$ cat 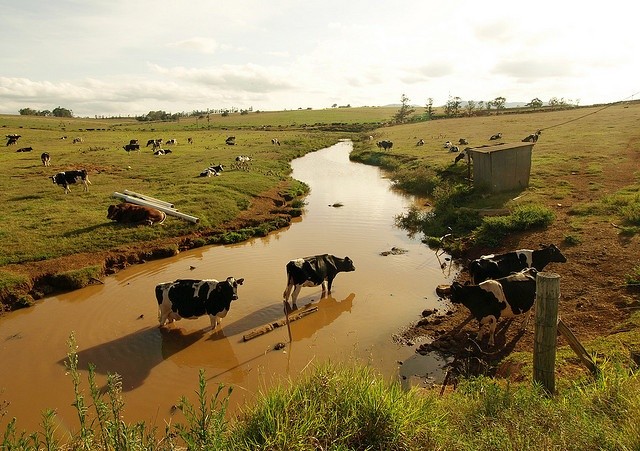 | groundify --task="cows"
[443,140,452,148]
[210,161,227,173]
[122,144,141,156]
[165,139,177,145]
[445,267,539,347]
[49,169,92,194]
[156,139,163,144]
[382,140,394,151]
[236,154,254,165]
[14,135,21,140]
[41,151,51,167]
[200,167,221,178]
[459,138,467,145]
[450,145,459,152]
[469,243,567,286]
[226,136,235,142]
[455,147,473,165]
[271,138,280,147]
[7,139,16,146]
[155,276,244,330]
[153,149,173,156]
[522,134,539,143]
[376,141,382,151]
[130,139,139,145]
[282,254,356,315]
[188,137,193,144]
[17,146,34,152]
[107,202,167,227]
[6,134,15,140]
[146,140,156,147]
[489,133,502,139]
[151,142,160,151]
[417,138,424,146]
[226,141,237,146]
